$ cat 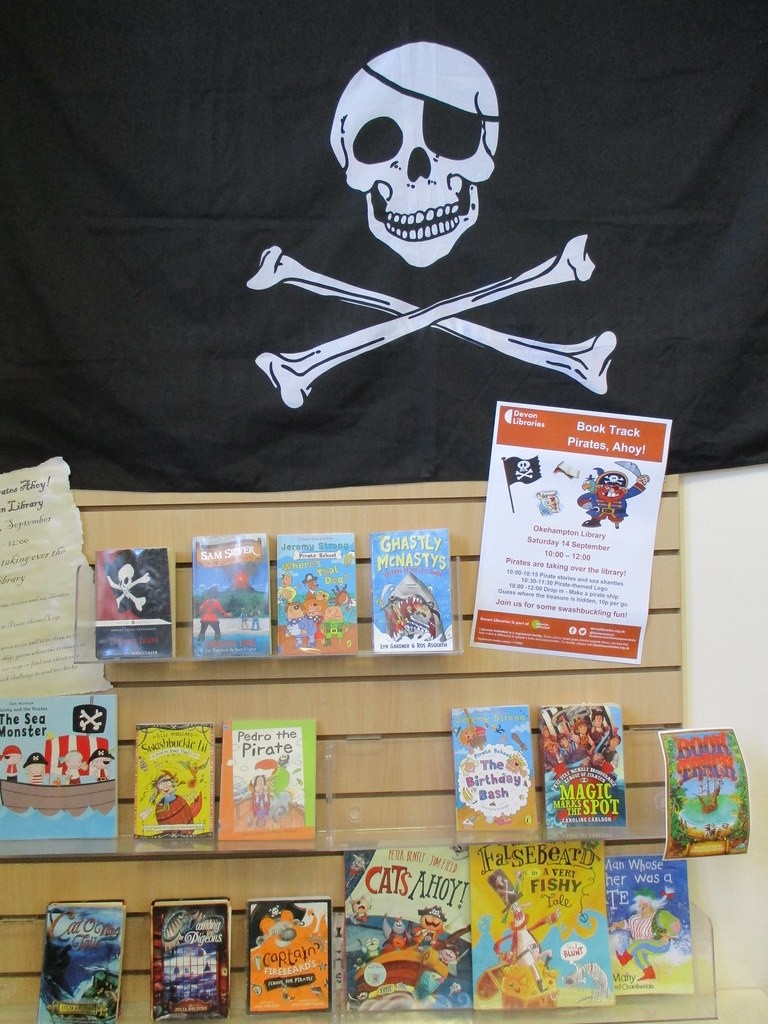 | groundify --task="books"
[133,721,214,840]
[94,547,177,662]
[0,693,119,840]
[658,726,751,862]
[343,846,472,1014]
[191,532,273,658]
[369,528,454,653]
[149,895,232,1021]
[277,533,359,655]
[218,718,315,840]
[469,841,616,1011]
[451,703,539,832]
[35,897,127,1024]
[603,852,694,996]
[245,895,335,1015]
[540,701,628,829]
